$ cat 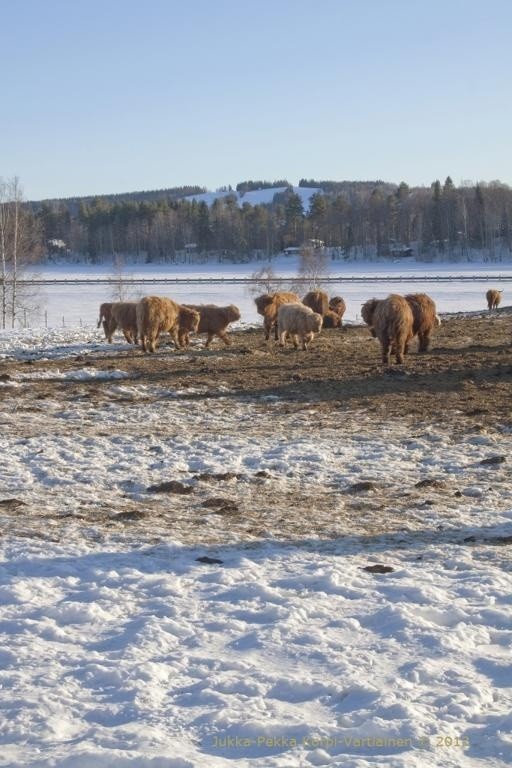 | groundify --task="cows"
[97,302,133,339]
[276,302,323,351]
[373,294,414,365]
[254,291,300,341]
[108,302,138,344]
[302,289,346,328]
[181,303,241,348]
[135,295,200,353]
[486,288,504,311]
[361,293,436,353]
